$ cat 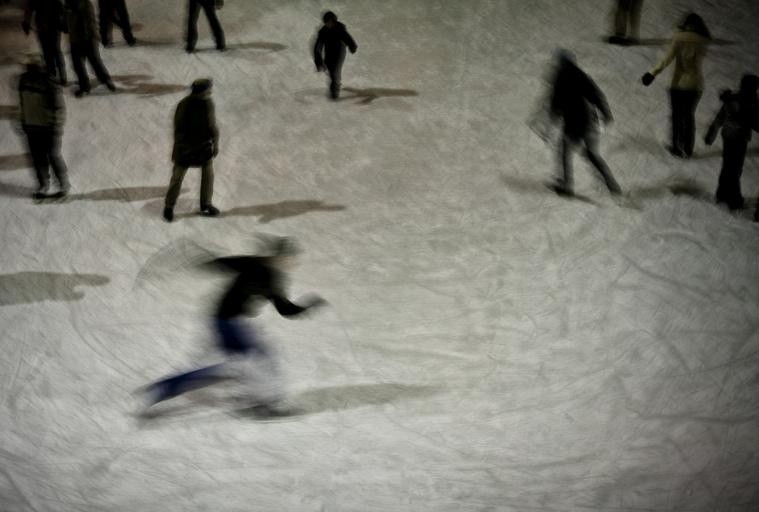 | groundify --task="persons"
[163,79,221,223]
[17,0,138,204]
[539,47,623,201]
[311,11,358,99]
[704,74,758,211]
[184,0,227,52]
[641,14,732,158]
[144,235,331,419]
[605,1,643,47]
